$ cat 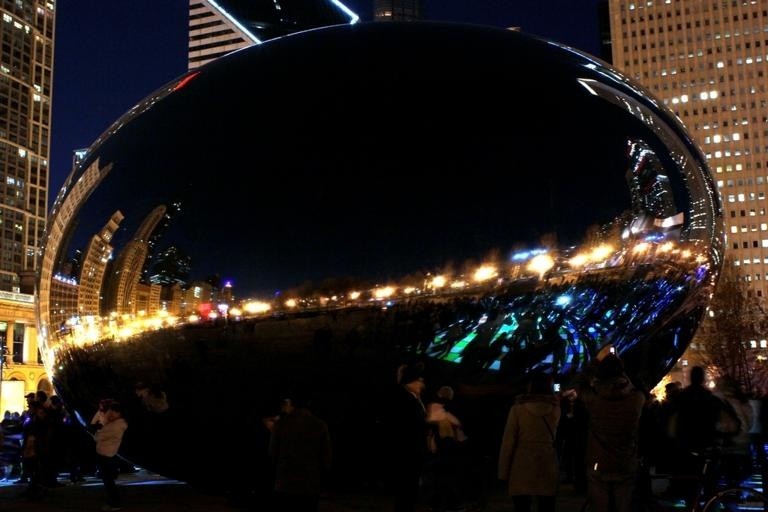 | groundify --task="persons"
[133,380,167,467]
[262,395,326,512]
[382,371,476,512]
[0,390,65,484]
[87,397,128,511]
